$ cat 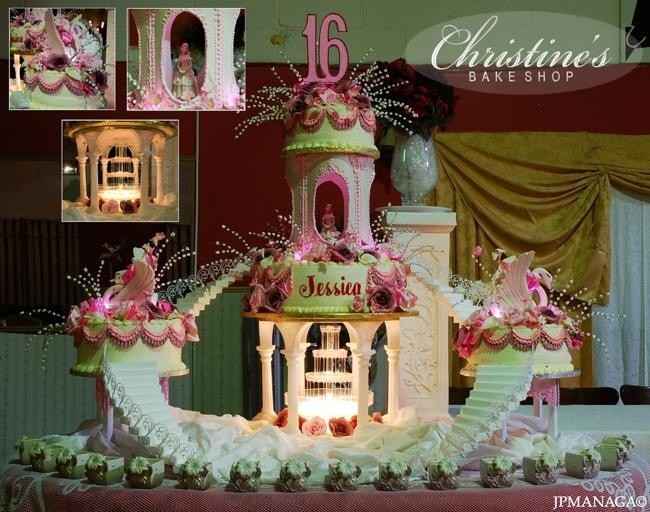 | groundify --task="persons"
[172,42,200,102]
[321,203,342,245]
